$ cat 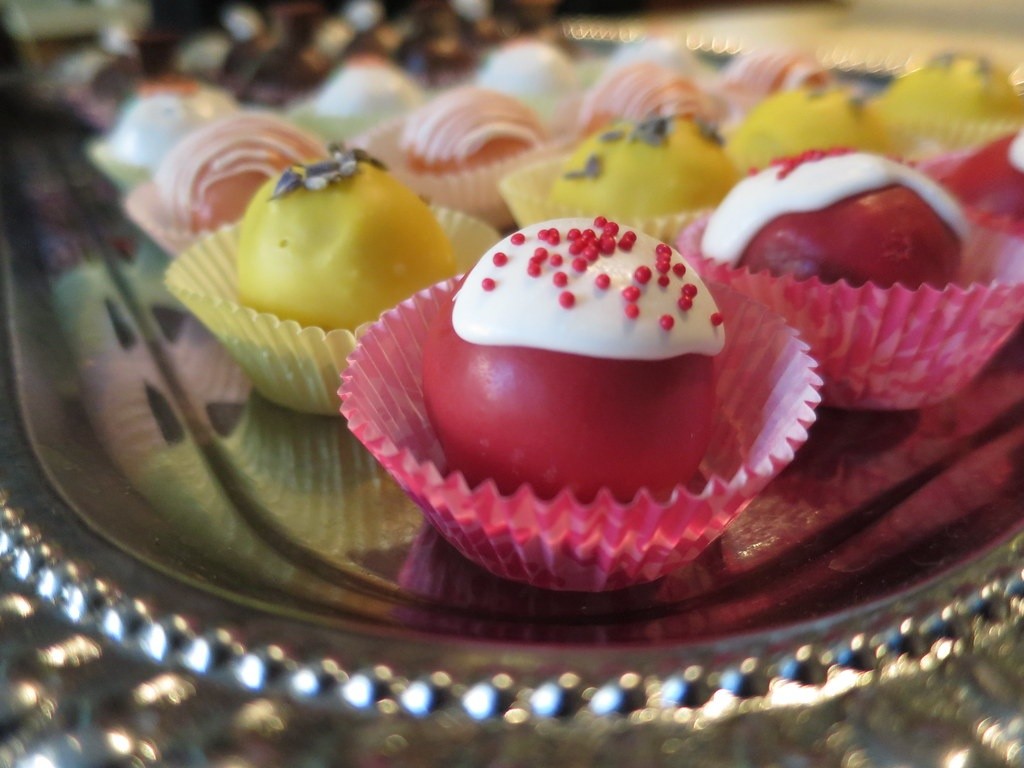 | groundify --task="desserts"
[84,33,1024,596]
